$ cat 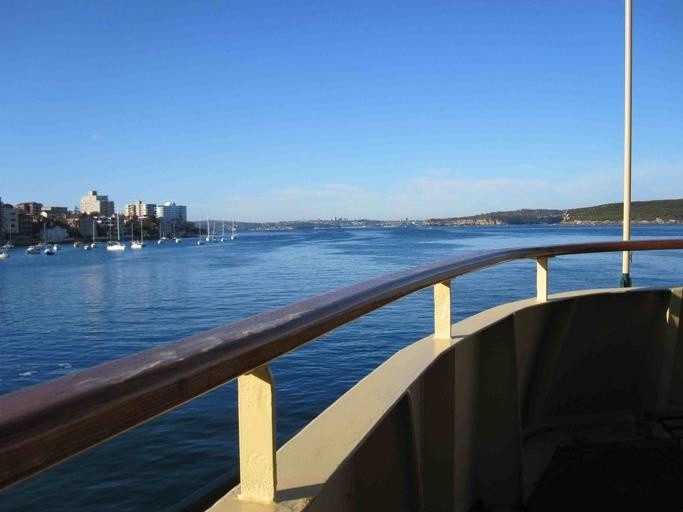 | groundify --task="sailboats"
[0,216,238,264]
